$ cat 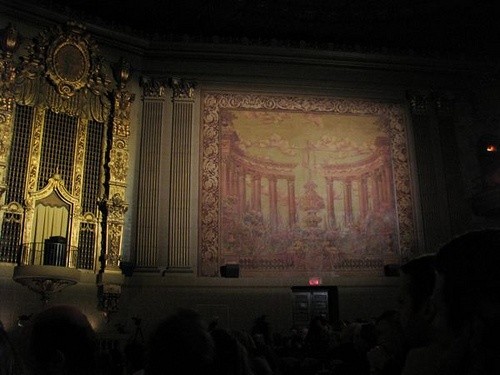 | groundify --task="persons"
[0,229,500,374]
